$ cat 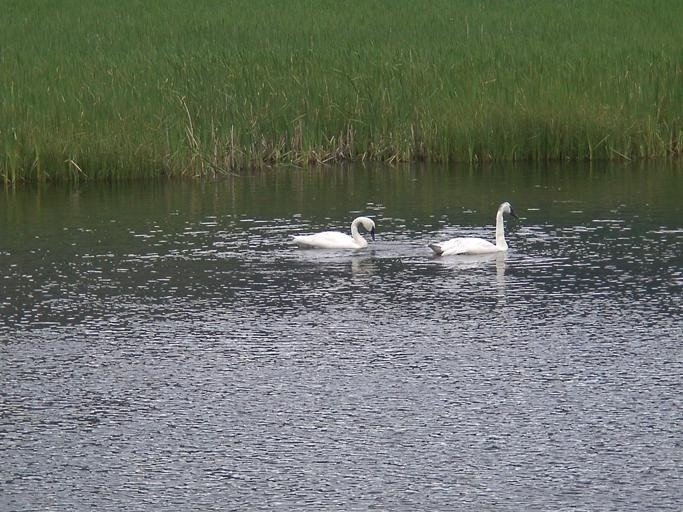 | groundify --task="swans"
[291,216,376,250]
[428,201,519,256]
